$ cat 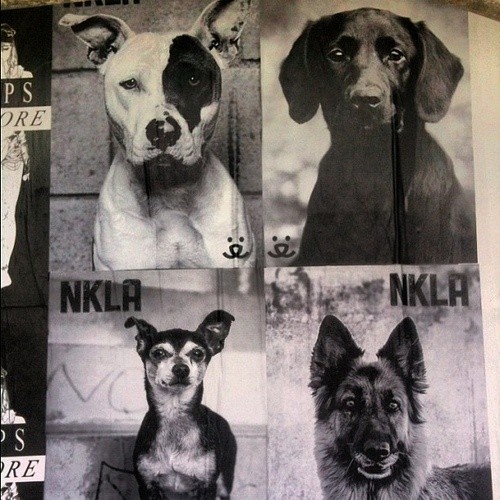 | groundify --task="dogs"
[278,7,478,267]
[58,0,257,268]
[124,309,238,500]
[307,315,493,500]
